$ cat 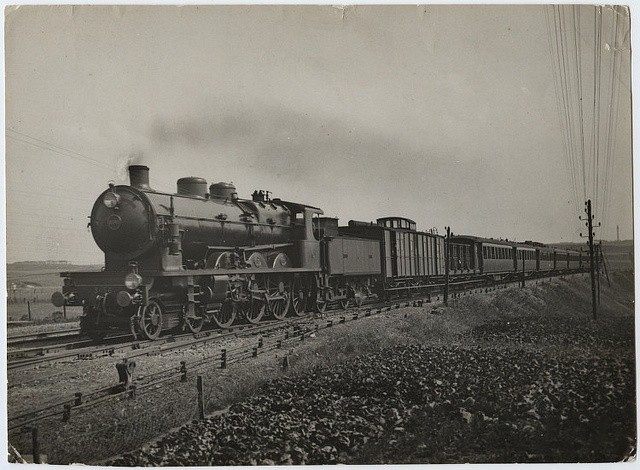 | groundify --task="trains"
[53,164,604,340]
